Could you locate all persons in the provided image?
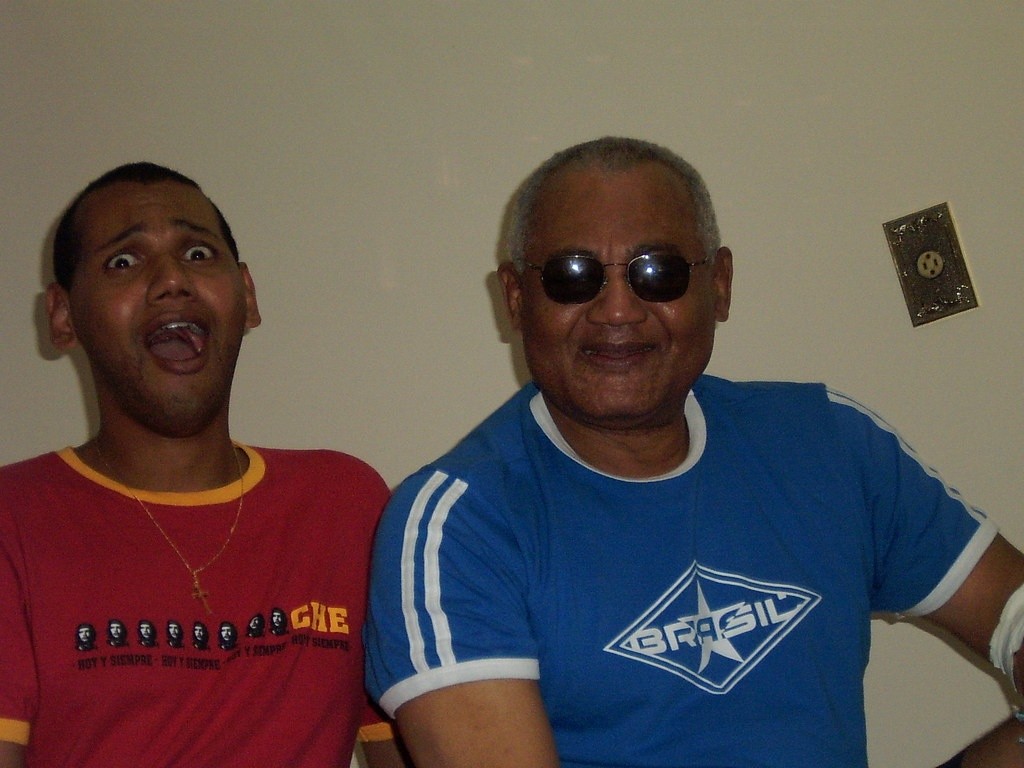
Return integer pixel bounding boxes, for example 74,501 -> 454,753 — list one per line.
1,159 -> 416,767
370,136 -> 1024,768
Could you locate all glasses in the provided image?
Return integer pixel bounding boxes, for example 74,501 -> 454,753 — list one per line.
522,249 -> 712,303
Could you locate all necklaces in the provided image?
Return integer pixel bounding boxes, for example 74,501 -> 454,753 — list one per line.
93,431 -> 247,614
